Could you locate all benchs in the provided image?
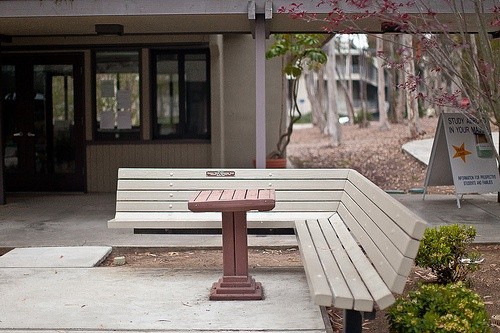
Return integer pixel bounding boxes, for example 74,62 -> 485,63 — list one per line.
108,165 -> 427,333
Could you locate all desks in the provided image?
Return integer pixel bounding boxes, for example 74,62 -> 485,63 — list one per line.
187,184 -> 276,301
